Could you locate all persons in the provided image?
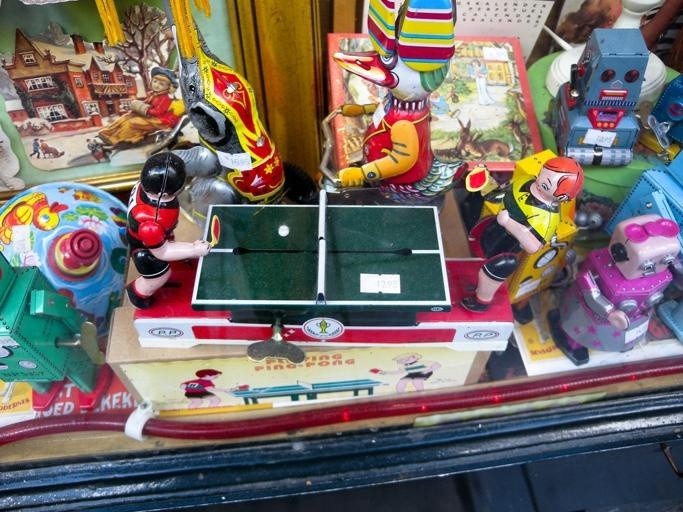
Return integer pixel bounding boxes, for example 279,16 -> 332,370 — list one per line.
459,156 -> 584,313
126,148 -> 210,309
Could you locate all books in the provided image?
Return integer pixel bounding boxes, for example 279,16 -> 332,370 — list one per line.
327,32 -> 543,189
